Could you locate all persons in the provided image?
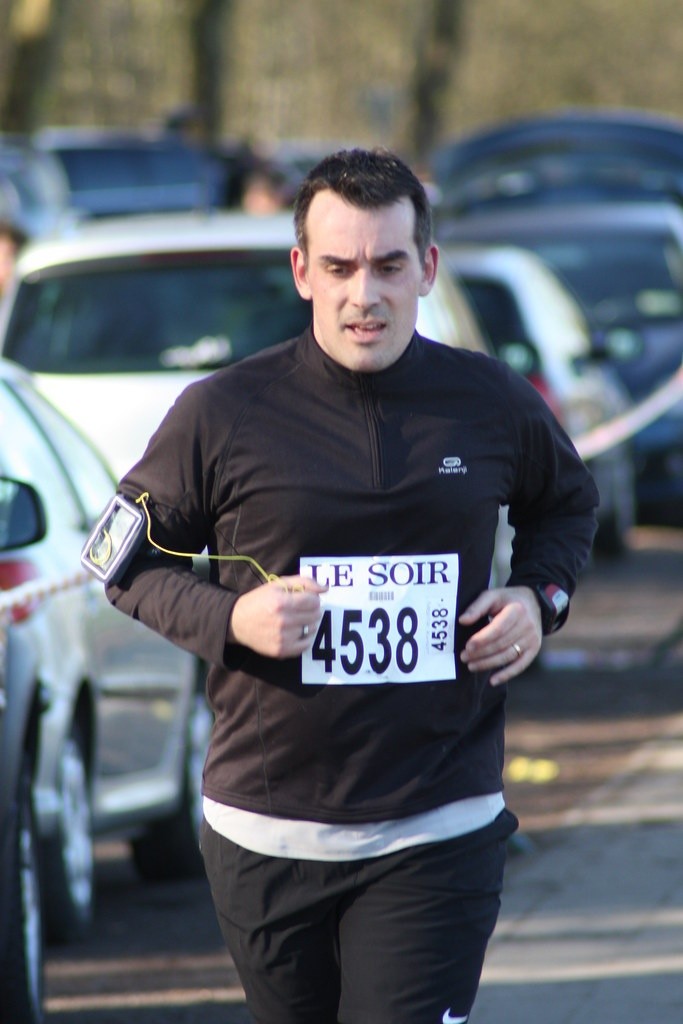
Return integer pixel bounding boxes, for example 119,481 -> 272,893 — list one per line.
79,148 -> 603,1024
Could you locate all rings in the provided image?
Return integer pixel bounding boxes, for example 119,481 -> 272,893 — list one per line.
513,644 -> 521,656
301,626 -> 310,639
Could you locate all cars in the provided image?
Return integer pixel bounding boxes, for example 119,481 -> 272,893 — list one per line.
1,475 -> 60,1024
0,105 -> 683,600
1,352 -> 216,948
